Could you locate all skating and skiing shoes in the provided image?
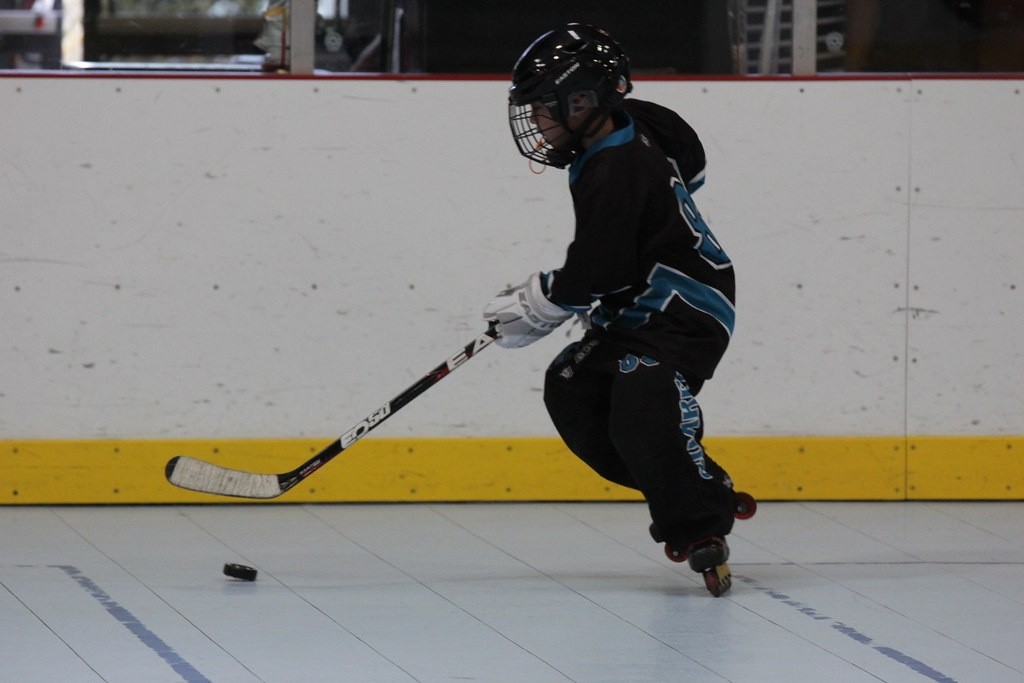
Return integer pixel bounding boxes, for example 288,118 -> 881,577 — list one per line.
686,536 -> 727,597
650,468 -> 756,562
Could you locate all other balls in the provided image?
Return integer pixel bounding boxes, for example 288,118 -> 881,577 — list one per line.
223,562 -> 258,582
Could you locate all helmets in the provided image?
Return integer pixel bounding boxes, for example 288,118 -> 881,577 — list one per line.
508,23 -> 633,169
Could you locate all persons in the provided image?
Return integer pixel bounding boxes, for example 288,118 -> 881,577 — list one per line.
481,22 -> 757,598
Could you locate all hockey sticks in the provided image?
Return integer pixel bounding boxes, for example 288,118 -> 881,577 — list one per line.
165,320 -> 498,499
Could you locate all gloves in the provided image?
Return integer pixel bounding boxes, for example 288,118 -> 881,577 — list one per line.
483,272 -> 574,348
